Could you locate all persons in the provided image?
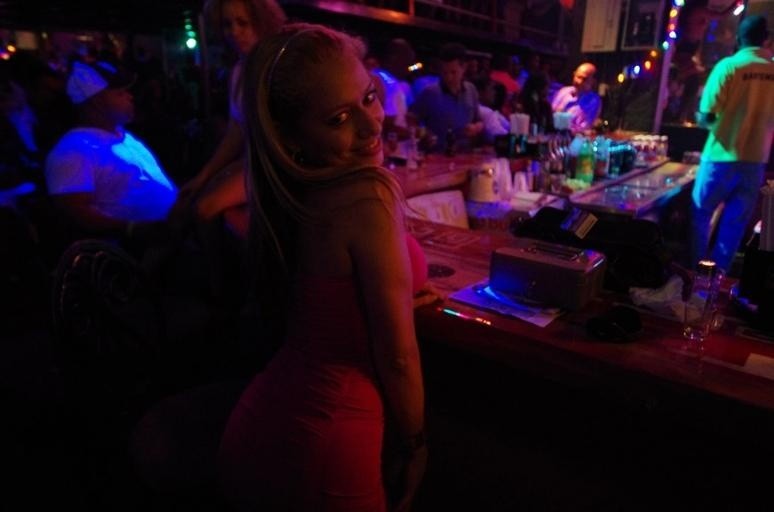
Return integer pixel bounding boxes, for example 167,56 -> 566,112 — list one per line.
217,20 -> 427,510
691,13 -> 772,272
43,58 -> 183,455
1,1 -> 704,350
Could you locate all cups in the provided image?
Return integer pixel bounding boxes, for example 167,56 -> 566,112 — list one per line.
684,302 -> 717,341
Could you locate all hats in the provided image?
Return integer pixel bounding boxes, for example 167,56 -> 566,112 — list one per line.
65,58 -> 137,105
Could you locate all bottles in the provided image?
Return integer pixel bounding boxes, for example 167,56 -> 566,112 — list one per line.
688,252 -> 743,334
522,133 -> 609,190
